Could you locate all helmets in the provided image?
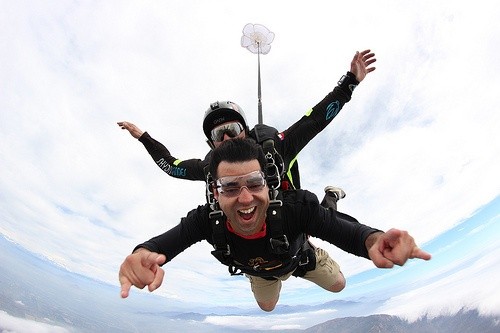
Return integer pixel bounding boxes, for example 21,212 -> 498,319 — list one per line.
204,99 -> 250,147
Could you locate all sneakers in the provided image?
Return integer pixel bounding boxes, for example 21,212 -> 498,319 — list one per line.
324,186 -> 346,199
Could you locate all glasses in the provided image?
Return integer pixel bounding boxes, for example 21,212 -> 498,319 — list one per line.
210,169 -> 269,198
209,123 -> 247,144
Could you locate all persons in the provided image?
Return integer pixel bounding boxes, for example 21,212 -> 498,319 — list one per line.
116,47 -> 376,210
119,138 -> 431,311
